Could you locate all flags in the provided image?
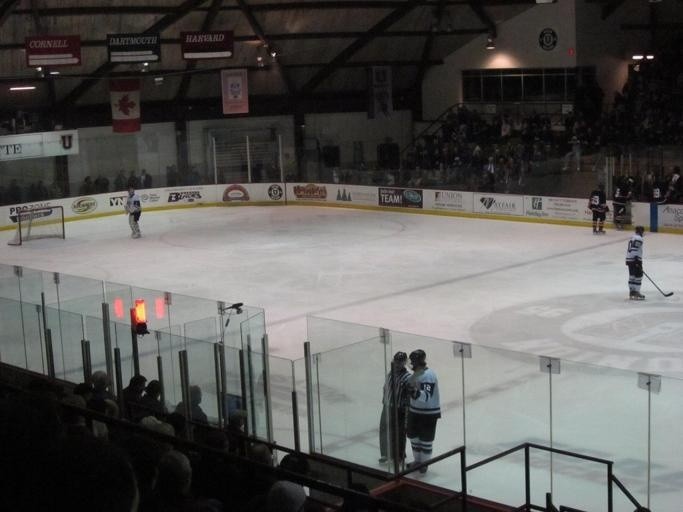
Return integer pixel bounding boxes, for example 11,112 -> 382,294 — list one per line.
220,69 -> 249,115
107,77 -> 140,135
179,29 -> 235,61
106,31 -> 163,66
23,35 -> 83,73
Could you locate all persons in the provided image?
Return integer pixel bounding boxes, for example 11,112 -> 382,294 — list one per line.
0,357 -> 315,512
123,187 -> 141,239
588,181 -> 610,237
0,154 -> 297,207
405,349 -> 441,474
625,225 -> 647,299
377,351 -> 411,464
613,174 -> 635,229
331,46 -> 683,205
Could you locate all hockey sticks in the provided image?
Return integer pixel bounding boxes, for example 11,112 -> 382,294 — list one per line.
642,269 -> 673,297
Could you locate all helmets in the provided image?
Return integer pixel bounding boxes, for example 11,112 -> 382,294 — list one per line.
395,351 -> 407,362
636,225 -> 644,234
597,182 -> 605,187
410,349 -> 426,364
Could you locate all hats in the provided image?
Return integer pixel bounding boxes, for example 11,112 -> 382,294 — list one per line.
229,410 -> 248,420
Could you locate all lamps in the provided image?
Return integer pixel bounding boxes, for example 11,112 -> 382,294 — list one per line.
483,35 -> 497,50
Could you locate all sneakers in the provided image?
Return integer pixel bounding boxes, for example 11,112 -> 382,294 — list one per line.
407,461 -> 427,474
378,456 -> 393,463
630,291 -> 645,298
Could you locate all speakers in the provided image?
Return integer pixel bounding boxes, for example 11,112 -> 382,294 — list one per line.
324,146 -> 340,166
377,143 -> 400,169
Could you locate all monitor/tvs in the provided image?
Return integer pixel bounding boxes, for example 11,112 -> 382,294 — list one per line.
221,392 -> 244,424
